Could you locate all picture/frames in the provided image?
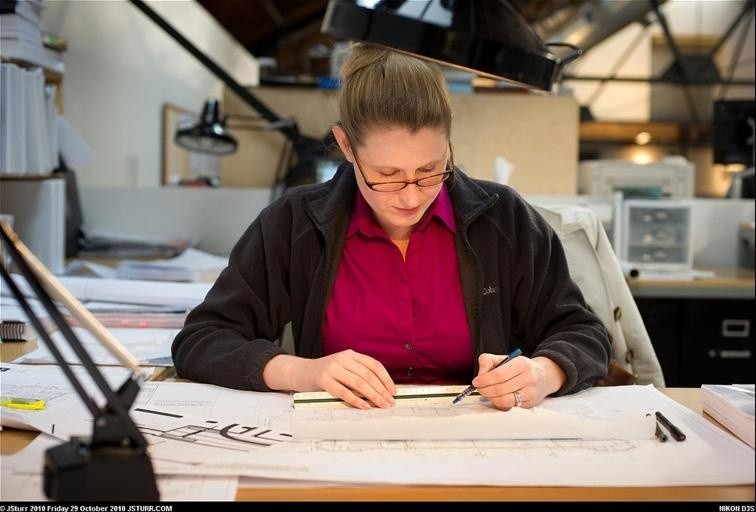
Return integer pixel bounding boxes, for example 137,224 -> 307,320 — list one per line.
621,199 -> 697,273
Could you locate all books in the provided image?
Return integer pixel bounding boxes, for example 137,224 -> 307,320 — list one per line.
59,301 -> 194,330
0,295 -> 62,343
1,0 -> 77,279
79,222 -> 203,262
109,259 -> 207,284
698,381 -> 756,450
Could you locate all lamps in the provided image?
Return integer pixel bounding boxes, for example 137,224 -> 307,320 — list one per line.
173,98 -> 301,203
318,0 -> 587,99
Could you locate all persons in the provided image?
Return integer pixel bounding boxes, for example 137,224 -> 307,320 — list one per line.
169,37 -> 615,413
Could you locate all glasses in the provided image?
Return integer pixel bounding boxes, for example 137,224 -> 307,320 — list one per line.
336,120 -> 454,193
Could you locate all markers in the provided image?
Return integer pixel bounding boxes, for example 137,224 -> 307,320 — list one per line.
0,396 -> 46,410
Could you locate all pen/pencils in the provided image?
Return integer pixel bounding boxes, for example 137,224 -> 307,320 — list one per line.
449,348 -> 522,407
646,412 -> 685,442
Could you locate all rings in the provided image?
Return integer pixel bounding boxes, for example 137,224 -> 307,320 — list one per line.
511,391 -> 522,408
